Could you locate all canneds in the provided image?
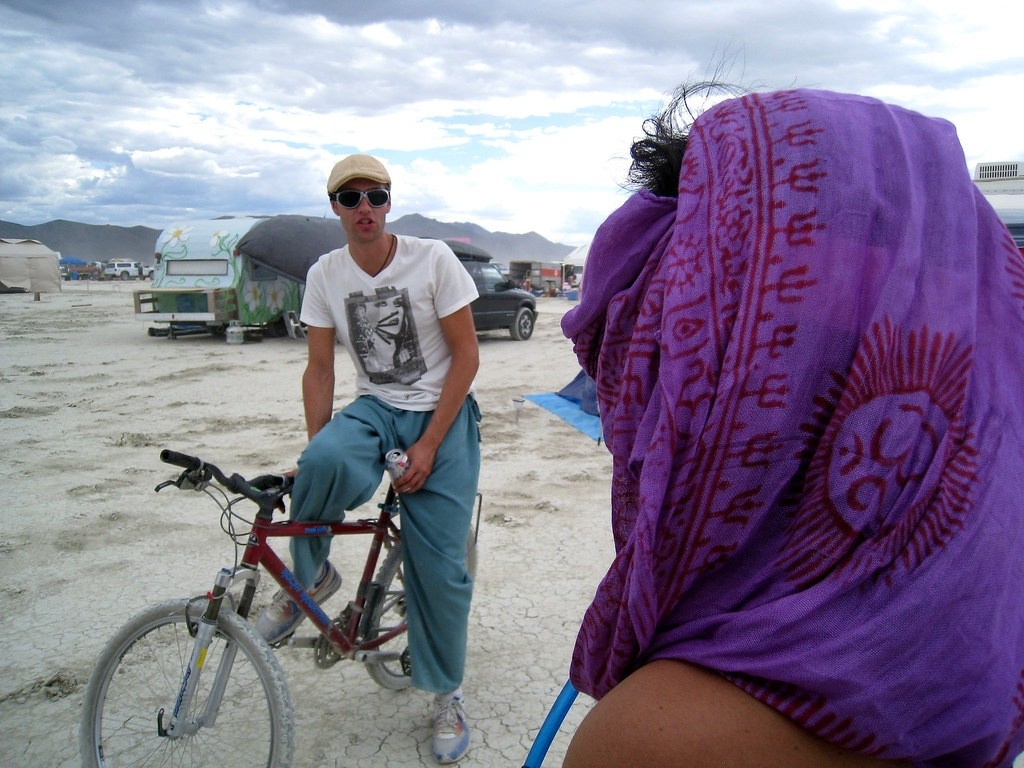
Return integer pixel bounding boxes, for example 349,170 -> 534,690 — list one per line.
384,449 -> 410,490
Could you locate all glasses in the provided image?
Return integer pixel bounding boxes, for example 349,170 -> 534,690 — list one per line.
329,189 -> 390,208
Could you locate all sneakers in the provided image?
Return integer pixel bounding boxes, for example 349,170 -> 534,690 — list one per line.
430,692 -> 470,763
252,558 -> 342,644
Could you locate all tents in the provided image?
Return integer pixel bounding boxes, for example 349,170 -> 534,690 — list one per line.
0,238 -> 63,294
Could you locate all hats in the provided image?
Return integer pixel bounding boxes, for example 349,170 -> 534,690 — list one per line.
327,154 -> 391,193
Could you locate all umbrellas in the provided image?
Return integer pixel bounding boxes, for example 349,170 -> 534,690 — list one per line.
563,239 -> 593,266
59,255 -> 87,265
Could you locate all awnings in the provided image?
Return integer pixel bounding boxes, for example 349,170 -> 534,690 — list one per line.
442,240 -> 494,263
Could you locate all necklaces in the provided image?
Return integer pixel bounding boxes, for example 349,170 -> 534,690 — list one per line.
372,234 -> 394,277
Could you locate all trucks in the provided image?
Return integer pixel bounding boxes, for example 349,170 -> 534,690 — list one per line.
510,260 -> 575,292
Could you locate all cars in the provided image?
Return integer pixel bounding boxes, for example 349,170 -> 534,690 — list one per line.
572,265 -> 583,286
104,262 -> 154,281
420,236 -> 538,340
482,262 -> 509,285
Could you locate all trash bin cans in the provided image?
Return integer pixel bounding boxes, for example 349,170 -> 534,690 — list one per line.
545,280 -> 558,297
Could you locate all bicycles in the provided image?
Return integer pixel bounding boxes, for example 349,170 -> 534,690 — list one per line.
78,450 -> 482,768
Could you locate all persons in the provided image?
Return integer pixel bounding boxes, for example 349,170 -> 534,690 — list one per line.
90,269 -> 98,281
564,87 -> 1023,767
64,261 -> 71,280
561,267 -> 574,282
138,263 -> 143,281
110,269 -> 117,280
252,153 -> 482,764
